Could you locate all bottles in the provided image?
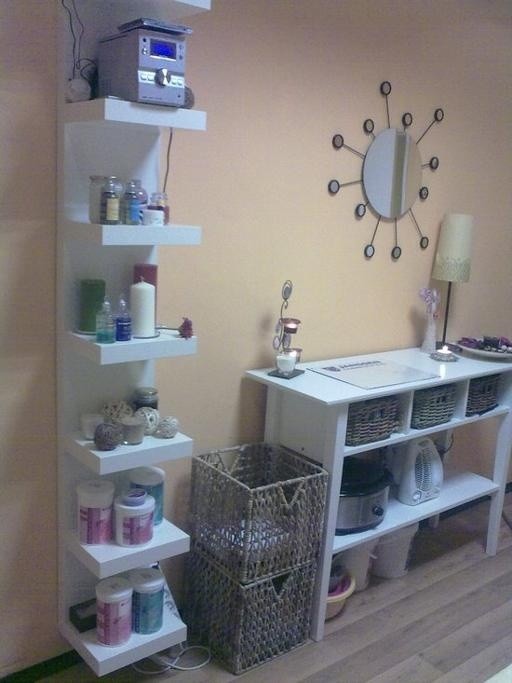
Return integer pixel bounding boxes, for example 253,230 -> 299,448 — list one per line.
114,299 -> 131,341
75,465 -> 166,549
128,568 -> 165,635
95,578 -> 133,647
94,295 -> 117,344
132,387 -> 159,412
99,175 -> 171,227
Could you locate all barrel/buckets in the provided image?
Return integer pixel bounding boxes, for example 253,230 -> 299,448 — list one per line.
333,538 -> 381,594
371,522 -> 422,580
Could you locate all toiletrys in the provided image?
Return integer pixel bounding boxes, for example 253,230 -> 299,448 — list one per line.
112,297 -> 132,342
95,301 -> 117,343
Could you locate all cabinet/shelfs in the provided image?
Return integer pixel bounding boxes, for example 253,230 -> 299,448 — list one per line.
54,0 -> 211,675
263,349 -> 510,644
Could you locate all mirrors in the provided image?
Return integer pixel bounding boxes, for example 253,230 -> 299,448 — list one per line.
361,127 -> 423,221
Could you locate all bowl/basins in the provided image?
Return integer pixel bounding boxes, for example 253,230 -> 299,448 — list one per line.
325,563 -> 356,623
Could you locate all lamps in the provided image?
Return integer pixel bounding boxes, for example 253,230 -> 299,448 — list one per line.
426,212 -> 479,362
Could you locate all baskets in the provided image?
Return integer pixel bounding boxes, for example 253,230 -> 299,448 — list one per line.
411,384 -> 458,430
466,374 -> 502,417
184,548 -> 318,676
344,395 -> 400,447
186,440 -> 329,585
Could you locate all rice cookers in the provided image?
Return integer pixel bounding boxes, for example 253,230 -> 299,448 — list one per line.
336,456 -> 395,536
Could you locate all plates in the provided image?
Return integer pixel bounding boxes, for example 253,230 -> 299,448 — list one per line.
267,369 -> 306,380
452,338 -> 512,359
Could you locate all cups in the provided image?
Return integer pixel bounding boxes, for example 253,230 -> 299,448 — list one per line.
134,263 -> 158,302
79,414 -> 104,439
78,278 -> 106,333
119,415 -> 145,445
276,354 -> 296,374
